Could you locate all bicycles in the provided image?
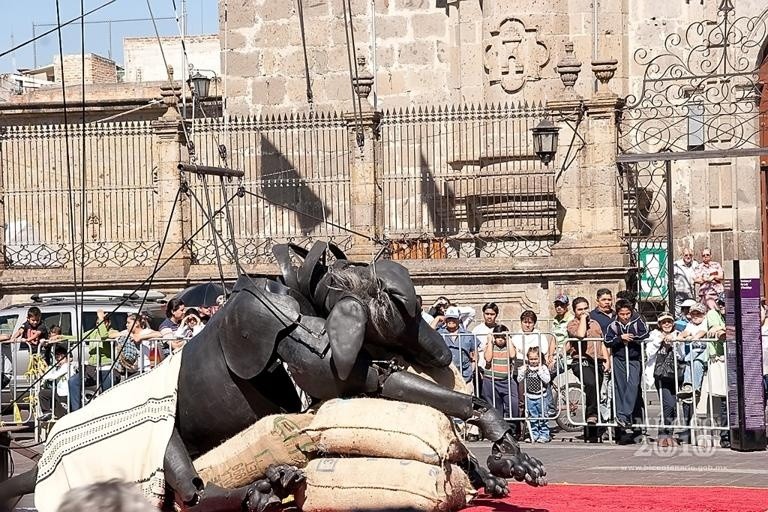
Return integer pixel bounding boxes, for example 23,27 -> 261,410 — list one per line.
549,358 -> 588,433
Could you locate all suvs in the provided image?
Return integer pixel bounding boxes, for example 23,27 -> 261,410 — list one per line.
0,288 -> 170,411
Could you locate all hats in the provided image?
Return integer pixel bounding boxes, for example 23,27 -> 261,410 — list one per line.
553,295 -> 568,303
445,306 -> 460,319
657,299 -> 706,322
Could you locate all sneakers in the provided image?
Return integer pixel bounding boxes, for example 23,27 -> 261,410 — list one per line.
524,437 -> 547,443
677,385 -> 693,399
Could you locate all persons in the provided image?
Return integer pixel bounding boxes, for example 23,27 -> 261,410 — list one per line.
416,248 -> 768,445
1,294 -> 228,422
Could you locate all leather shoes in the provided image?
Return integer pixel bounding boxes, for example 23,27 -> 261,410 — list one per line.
587,417 -> 596,424
37,414 -> 51,421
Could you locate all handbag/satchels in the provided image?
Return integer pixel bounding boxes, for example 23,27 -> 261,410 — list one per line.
654,340 -> 685,382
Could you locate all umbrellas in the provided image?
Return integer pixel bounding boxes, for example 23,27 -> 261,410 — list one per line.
173,277 -> 231,306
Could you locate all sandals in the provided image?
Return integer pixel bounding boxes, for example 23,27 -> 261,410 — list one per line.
615,416 -> 627,428
